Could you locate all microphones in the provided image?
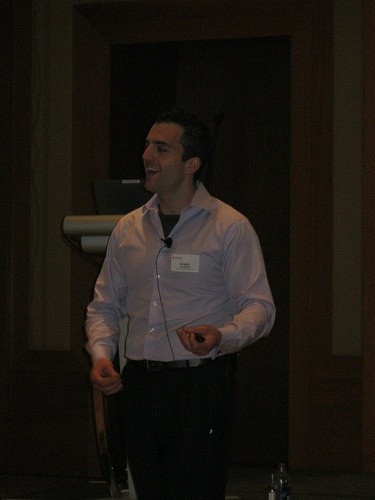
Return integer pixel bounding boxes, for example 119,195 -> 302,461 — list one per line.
161,238 -> 173,248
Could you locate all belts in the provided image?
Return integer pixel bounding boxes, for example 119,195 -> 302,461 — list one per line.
132,360 -> 187,369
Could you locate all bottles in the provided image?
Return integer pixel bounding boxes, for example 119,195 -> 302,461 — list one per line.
271,463 -> 288,500
268,489 -> 276,500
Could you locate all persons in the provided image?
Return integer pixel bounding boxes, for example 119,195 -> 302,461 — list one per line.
84,112 -> 277,500
